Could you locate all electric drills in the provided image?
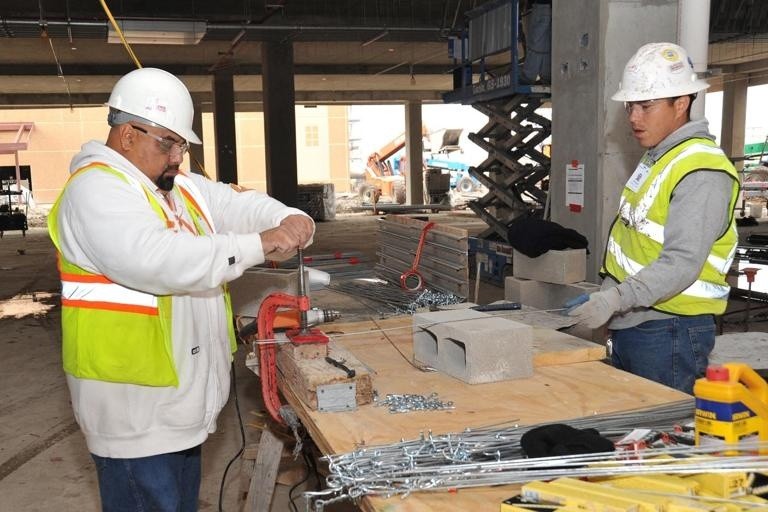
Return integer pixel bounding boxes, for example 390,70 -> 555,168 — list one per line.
240,310 -> 357,338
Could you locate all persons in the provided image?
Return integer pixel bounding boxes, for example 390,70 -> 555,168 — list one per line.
45,67 -> 317,512
565,40 -> 741,399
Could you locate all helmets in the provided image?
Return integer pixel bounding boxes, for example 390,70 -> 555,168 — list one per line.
610,42 -> 711,102
104,68 -> 202,146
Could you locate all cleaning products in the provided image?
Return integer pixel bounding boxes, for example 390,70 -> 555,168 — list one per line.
693,362 -> 768,457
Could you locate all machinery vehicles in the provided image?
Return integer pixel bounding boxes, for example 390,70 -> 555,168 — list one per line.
392,151 -> 483,193
360,120 -> 466,215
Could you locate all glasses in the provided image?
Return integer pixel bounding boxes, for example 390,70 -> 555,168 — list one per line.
624,99 -> 673,114
132,125 -> 190,155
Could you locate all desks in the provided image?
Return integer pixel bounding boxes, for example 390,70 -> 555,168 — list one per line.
223,277 -> 700,512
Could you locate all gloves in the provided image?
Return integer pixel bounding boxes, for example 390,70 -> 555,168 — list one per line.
567,286 -> 621,329
